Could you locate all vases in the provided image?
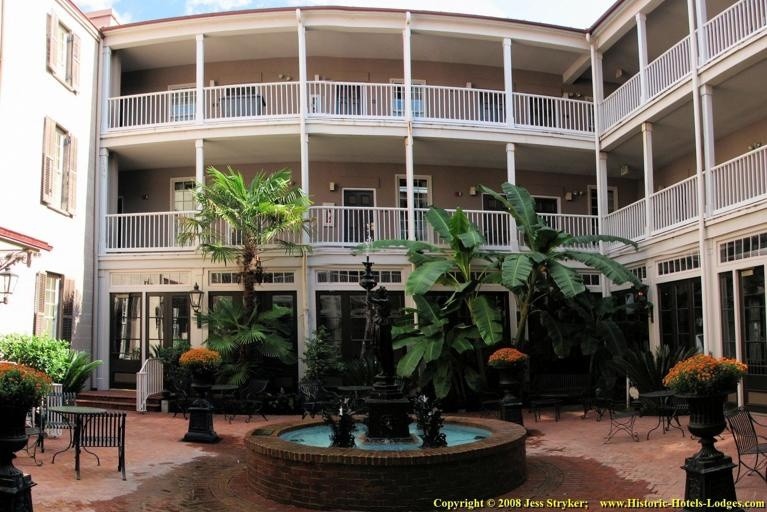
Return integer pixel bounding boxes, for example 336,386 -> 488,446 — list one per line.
183,368 -> 223,444
0,414 -> 33,511
496,361 -> 528,434
674,393 -> 745,512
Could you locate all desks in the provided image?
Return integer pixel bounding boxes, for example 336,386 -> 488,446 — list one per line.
338,385 -> 374,408
639,389 -> 685,440
209,384 -> 239,419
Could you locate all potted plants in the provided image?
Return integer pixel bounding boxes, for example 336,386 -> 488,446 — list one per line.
0,362 -> 50,414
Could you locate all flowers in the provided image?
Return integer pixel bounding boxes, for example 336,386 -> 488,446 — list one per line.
663,352 -> 748,396
178,348 -> 222,374
488,345 -> 529,366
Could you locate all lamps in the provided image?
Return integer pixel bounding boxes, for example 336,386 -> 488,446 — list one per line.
0,247 -> 41,304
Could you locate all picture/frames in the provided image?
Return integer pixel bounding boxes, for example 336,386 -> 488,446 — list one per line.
321,202 -> 336,228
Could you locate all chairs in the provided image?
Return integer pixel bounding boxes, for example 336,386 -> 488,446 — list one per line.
171,376 -> 194,420
298,382 -> 344,420
725,405 -> 767,485
26,390 -> 127,481
227,379 -> 270,423
531,367 -> 640,442
